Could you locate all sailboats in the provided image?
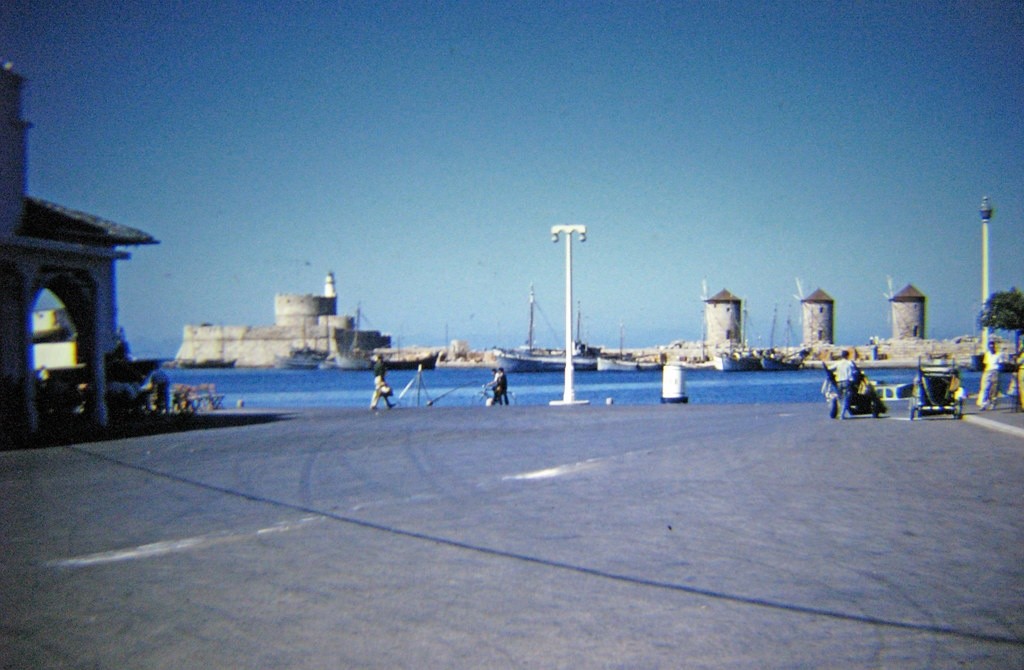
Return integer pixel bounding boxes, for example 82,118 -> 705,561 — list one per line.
713,295 -> 737,371
735,299 -> 762,370
334,303 -> 372,371
760,302 -> 811,370
493,284 -> 596,371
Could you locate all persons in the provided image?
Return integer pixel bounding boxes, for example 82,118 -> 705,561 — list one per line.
1013,334 -> 1024,412
150,366 -> 171,414
821,350 -> 858,421
486,366 -> 511,406
976,342 -> 1003,412
371,354 -> 397,409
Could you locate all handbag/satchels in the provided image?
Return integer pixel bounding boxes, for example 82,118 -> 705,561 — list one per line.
379,386 -> 393,397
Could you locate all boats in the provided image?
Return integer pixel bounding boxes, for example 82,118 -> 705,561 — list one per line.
680,361 -> 714,368
871,383 -> 914,401
596,358 -> 636,372
638,361 -> 660,370
179,357 -> 236,368
373,351 -> 437,369
276,344 -> 330,369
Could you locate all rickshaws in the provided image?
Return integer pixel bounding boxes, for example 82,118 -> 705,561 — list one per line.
908,355 -> 964,421
822,358 -> 884,418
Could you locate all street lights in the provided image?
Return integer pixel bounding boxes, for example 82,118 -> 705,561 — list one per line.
979,195 -> 991,352
550,223 -> 587,403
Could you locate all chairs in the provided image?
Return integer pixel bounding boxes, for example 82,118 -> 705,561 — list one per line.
173,384 -> 225,410
